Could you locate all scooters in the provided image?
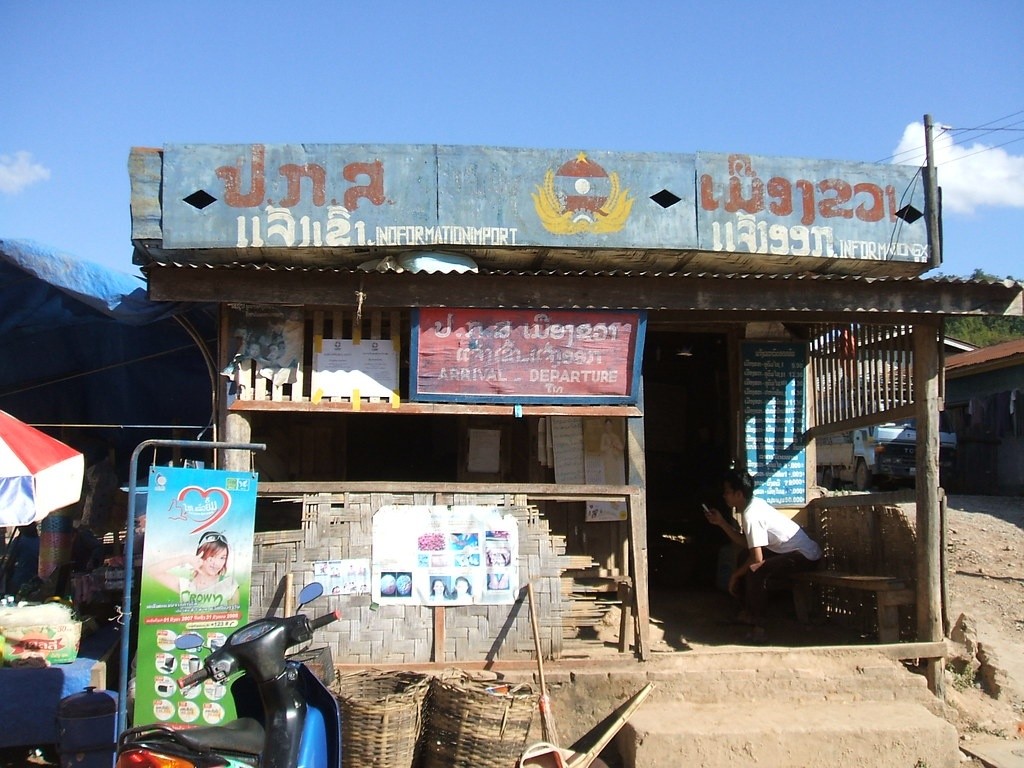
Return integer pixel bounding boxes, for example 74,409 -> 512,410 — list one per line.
114,580 -> 341,768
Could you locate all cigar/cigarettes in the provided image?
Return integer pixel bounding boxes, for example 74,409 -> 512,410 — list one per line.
702,503 -> 712,514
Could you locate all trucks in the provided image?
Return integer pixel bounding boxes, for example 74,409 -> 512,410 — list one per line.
816,398 -> 958,491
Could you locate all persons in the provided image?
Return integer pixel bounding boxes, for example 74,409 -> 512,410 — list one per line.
428,576 -> 475,600
147,531 -> 242,620
701,470 -> 826,644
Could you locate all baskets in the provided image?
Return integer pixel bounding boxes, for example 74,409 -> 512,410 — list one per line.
285,646 -> 335,687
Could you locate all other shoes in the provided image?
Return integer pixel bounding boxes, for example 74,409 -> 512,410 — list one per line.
730,613 -> 769,644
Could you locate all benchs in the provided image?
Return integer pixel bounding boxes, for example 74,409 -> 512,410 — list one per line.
775,570 -> 916,645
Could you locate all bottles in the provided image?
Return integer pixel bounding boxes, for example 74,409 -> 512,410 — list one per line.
64,595 -> 81,622
0,593 -> 17,607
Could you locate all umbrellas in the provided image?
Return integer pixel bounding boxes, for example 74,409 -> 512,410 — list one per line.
0,406 -> 85,531
